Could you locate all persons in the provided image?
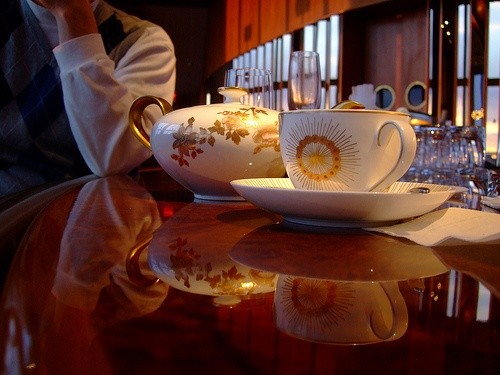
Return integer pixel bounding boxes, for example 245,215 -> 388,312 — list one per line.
0,1 -> 177,189
4,177 -> 170,375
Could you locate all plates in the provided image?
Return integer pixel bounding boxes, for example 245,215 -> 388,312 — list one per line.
230,221 -> 454,283
230,177 -> 469,229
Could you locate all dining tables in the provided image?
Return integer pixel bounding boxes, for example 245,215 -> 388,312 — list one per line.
0,167 -> 500,375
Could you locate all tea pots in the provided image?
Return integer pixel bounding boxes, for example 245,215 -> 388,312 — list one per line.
129,86 -> 287,206
127,200 -> 284,308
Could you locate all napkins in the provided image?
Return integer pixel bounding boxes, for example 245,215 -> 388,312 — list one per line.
361,208 -> 500,247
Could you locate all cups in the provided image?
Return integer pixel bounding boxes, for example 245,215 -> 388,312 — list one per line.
289,50 -> 322,110
274,275 -> 412,345
224,68 -> 274,111
277,109 -> 418,192
414,125 -> 484,210
